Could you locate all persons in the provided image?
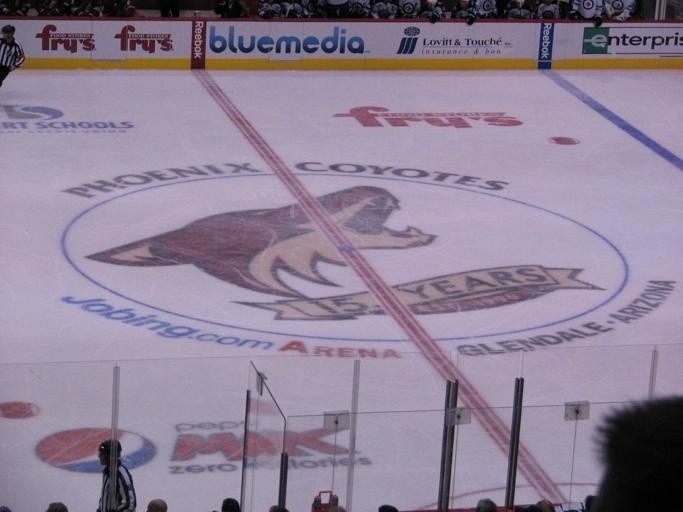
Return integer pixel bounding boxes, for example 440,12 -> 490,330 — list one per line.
145,498 -> 167,511
220,496 -> 239,510
0,25 -> 25,88
0,0 -> 683,26
96,439 -> 137,512
585,391 -> 682,510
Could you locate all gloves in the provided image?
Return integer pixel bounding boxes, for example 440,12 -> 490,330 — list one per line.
430,13 -> 440,24
592,16 -> 603,28
466,13 -> 477,26
605,4 -> 615,19
259,9 -> 278,20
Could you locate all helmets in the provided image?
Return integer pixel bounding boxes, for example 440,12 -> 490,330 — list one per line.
2,25 -> 16,34
100,438 -> 122,458
312,490 -> 338,510
